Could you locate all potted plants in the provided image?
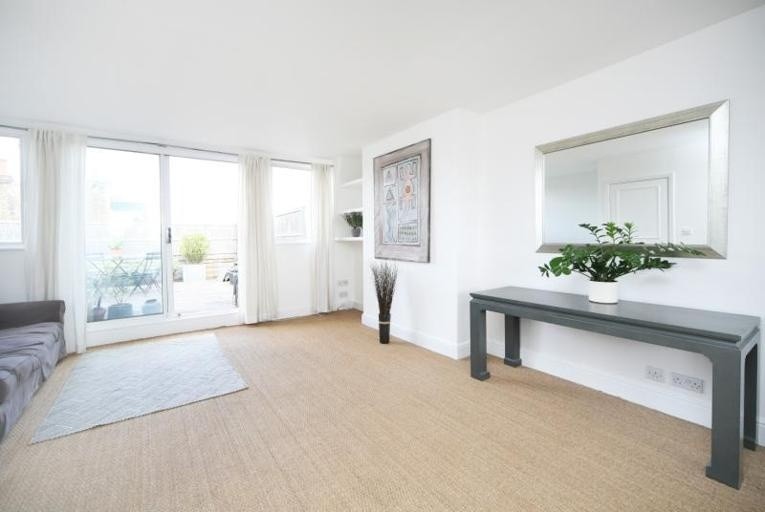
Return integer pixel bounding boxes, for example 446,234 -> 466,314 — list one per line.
89,278 -> 106,321
340,211 -> 363,237
105,273 -> 133,319
538,219 -> 708,305
178,233 -> 210,290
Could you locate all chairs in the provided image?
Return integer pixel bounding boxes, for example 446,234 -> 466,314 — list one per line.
129,251 -> 161,297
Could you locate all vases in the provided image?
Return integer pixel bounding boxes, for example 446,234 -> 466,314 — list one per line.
378,313 -> 390,345
141,299 -> 161,315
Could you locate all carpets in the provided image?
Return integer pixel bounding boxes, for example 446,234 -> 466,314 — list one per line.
26,330 -> 250,445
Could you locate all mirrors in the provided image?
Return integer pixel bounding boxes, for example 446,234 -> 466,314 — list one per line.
533,99 -> 728,260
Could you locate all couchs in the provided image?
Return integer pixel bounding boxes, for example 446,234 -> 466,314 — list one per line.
0,301 -> 67,442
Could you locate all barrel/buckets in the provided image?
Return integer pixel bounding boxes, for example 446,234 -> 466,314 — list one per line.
182,264 -> 207,289
87,308 -> 106,321
107,302 -> 133,320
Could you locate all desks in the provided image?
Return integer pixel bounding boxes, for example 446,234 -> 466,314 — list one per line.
467,285 -> 761,490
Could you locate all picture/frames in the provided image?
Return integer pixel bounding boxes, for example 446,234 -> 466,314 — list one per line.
372,138 -> 431,265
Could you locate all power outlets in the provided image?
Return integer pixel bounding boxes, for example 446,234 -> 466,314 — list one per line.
670,371 -> 704,394
643,365 -> 667,383
